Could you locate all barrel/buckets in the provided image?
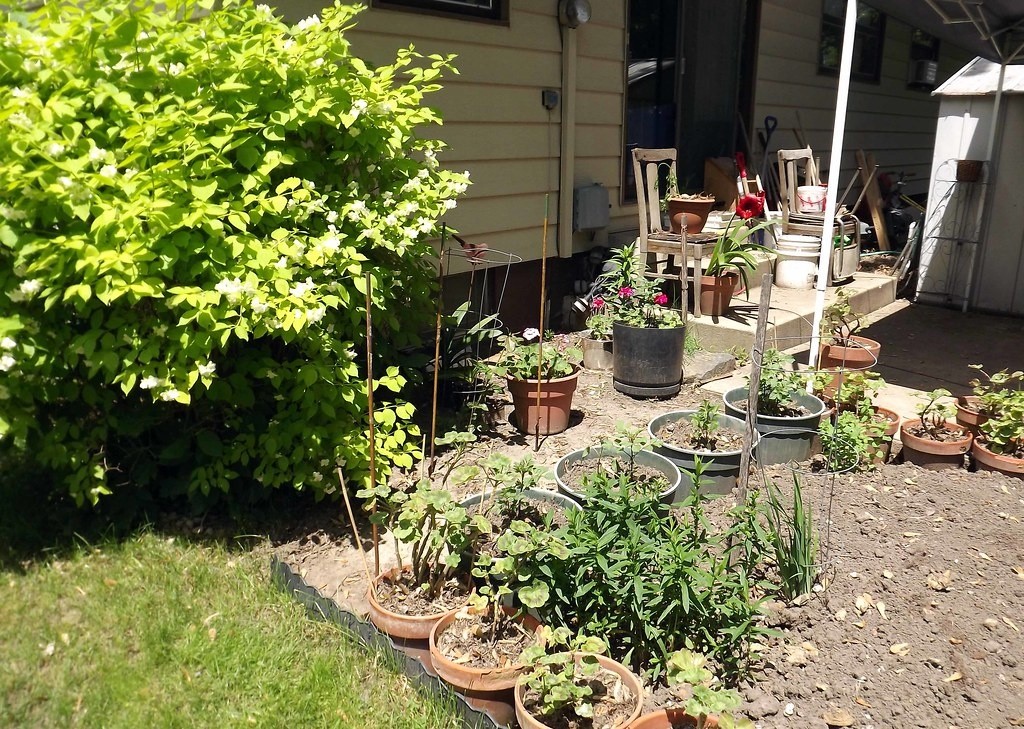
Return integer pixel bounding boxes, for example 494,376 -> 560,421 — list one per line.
798,185 -> 827,212
774,233 -> 822,288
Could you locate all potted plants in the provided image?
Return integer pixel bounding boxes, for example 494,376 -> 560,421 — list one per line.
651,163 -> 714,233
368,290 -> 1024,729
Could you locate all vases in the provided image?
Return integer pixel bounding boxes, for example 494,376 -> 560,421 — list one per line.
612,319 -> 687,395
578,330 -> 614,375
699,271 -> 738,317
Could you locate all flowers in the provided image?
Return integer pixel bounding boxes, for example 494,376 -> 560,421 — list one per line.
704,193 -> 781,300
583,300 -> 614,340
620,289 -> 682,327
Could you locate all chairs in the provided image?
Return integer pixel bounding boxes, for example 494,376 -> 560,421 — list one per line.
633,148 -> 728,318
778,148 -> 860,286
